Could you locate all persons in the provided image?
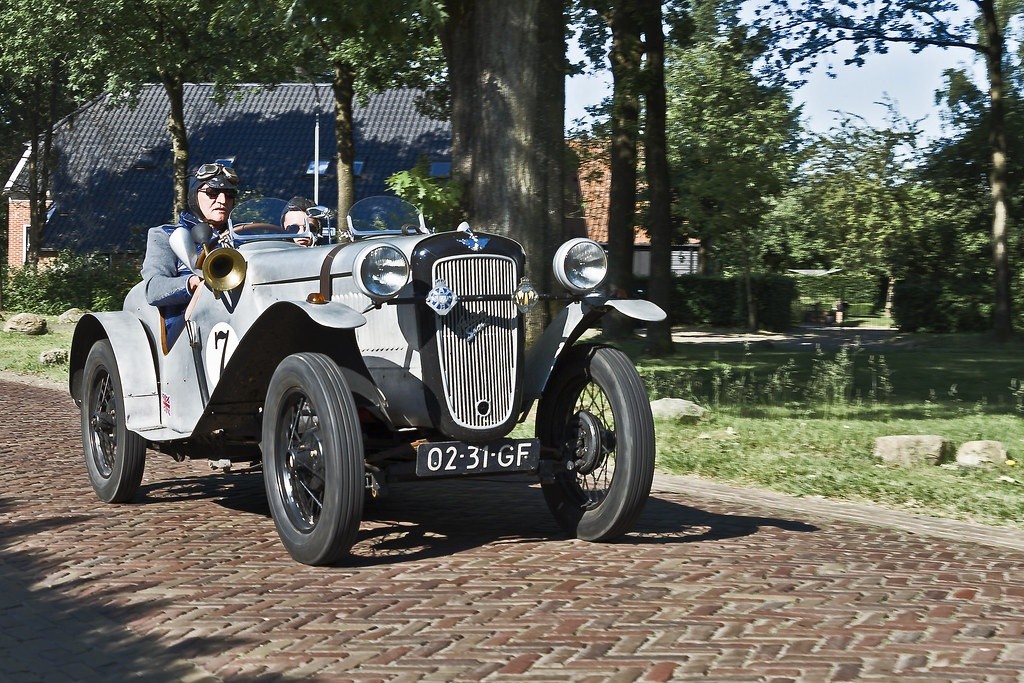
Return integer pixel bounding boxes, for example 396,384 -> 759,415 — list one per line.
140,162 -> 239,352
280,195 -> 325,247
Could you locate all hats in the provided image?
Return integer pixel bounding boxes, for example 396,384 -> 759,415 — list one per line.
188,173 -> 237,219
281,198 -> 322,240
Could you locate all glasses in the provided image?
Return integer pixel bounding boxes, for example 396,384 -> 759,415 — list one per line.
195,164 -> 240,184
197,188 -> 238,199
286,223 -> 317,234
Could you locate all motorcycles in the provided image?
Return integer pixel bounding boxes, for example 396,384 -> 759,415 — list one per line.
67,196 -> 668,566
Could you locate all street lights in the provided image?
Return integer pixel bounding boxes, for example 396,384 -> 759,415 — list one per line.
293,66 -> 322,207
